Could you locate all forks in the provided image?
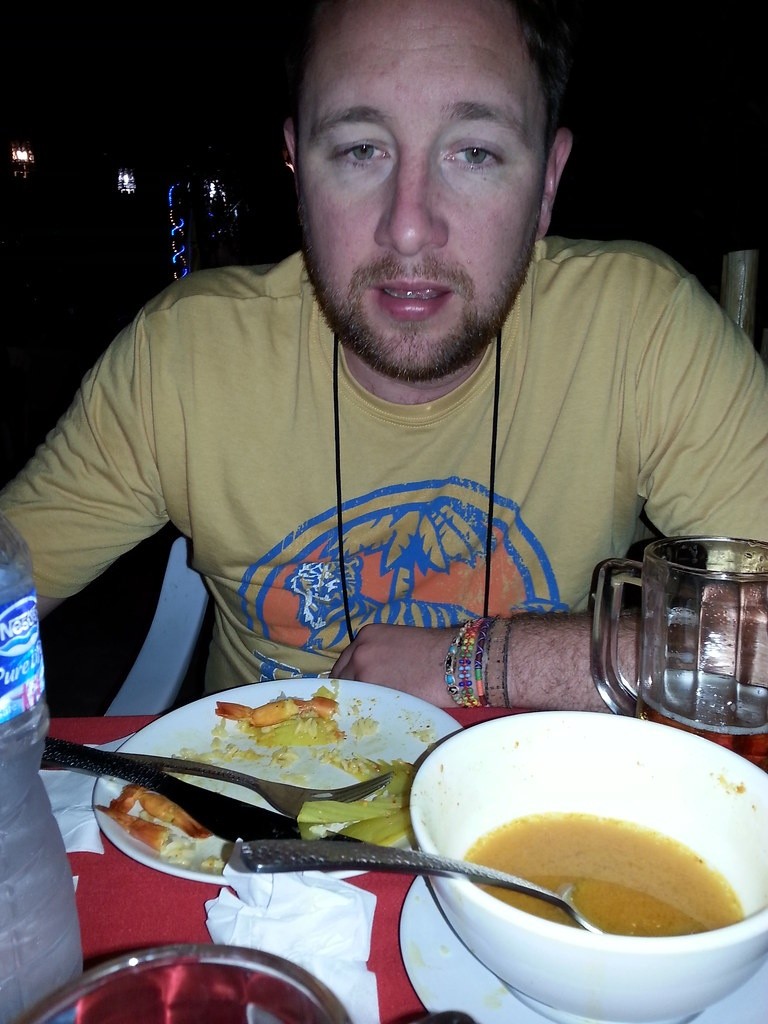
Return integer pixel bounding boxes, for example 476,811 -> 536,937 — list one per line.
40,751 -> 394,818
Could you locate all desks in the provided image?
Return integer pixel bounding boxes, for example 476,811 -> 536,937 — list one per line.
39,706 -> 768,1024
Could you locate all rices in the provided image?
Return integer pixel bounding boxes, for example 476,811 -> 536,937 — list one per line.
139,680 -> 438,869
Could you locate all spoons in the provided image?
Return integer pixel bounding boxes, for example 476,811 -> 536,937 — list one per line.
222,837 -> 607,935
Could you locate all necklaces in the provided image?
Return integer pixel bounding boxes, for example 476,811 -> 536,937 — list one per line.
332,333 -> 501,644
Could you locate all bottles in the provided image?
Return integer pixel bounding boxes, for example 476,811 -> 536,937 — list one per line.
0,508 -> 83,1024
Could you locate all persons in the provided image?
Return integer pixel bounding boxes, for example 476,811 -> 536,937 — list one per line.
0,1 -> 768,712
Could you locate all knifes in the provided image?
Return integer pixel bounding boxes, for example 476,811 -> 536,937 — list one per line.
42,737 -> 364,843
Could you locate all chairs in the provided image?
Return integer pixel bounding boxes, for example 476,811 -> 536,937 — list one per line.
104,536 -> 210,717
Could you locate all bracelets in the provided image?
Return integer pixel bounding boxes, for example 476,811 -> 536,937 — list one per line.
442,616 -> 511,708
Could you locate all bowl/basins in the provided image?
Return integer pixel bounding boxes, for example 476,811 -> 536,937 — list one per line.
410,712 -> 768,1024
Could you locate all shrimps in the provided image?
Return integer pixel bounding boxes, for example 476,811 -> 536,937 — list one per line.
94,784 -> 212,852
215,696 -> 340,727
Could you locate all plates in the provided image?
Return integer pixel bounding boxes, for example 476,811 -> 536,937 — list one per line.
399,872 -> 768,1024
92,678 -> 466,887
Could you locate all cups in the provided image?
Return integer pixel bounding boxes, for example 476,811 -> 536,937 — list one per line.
30,943 -> 481,1024
588,534 -> 768,771
